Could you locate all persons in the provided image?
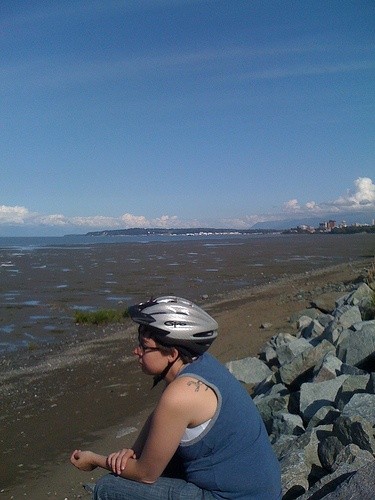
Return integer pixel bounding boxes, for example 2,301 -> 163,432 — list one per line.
69,296 -> 284,500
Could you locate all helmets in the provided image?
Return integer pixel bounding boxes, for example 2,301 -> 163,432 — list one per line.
128,295 -> 219,354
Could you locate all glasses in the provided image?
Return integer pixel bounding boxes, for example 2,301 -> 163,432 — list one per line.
138,339 -> 172,356
